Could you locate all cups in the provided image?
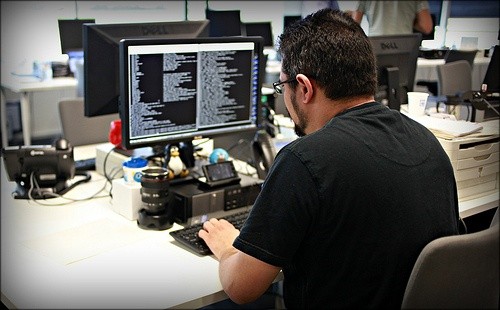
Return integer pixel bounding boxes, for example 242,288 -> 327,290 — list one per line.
407,92 -> 430,118
121,158 -> 171,214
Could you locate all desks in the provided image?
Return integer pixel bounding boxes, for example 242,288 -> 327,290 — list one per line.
1,128 -> 499,309
413,53 -> 490,123
0,65 -> 78,146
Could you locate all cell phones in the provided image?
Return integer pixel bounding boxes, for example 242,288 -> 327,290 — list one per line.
202,161 -> 238,183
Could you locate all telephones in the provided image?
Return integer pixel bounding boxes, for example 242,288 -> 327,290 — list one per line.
2,137 -> 76,199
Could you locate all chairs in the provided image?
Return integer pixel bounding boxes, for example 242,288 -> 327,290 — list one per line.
401,208 -> 499,310
58,99 -> 119,146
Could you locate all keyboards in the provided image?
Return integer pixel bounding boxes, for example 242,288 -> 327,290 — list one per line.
76,158 -> 96,171
169,211 -> 250,256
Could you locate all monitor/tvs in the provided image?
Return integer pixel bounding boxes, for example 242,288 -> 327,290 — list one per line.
367,32 -> 422,111
59,19 -> 274,183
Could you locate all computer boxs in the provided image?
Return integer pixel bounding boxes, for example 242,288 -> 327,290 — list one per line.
162,158 -> 265,227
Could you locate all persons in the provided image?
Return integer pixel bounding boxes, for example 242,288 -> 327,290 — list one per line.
199,7 -> 459,310
344,0 -> 433,35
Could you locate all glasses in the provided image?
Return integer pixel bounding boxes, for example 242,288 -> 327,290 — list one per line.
273,77 -> 296,94
